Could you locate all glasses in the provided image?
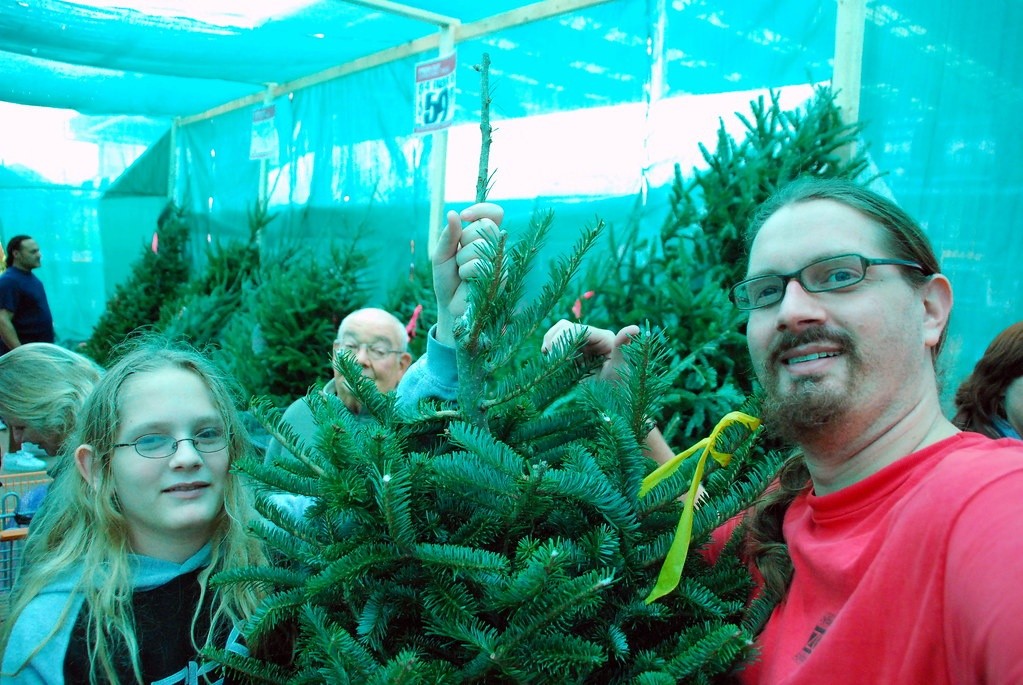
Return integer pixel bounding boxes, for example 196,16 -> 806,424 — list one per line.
338,341 -> 404,359
113,427 -> 235,459
727,253 -> 929,310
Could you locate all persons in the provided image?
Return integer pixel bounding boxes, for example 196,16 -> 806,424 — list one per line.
264,307 -> 411,492
951,321 -> 1023,441
1,203 -> 505,684
0,342 -> 110,460
0,235 -> 57,351
539,173 -> 1023,683
